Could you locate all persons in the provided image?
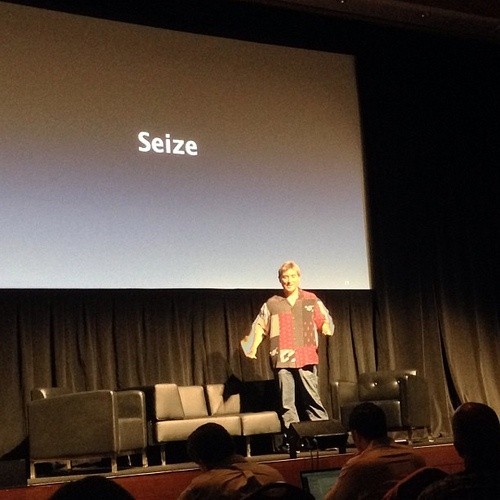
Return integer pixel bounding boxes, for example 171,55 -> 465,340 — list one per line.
326,402 -> 425,500
418,402 -> 500,500
48,477 -> 136,500
240,262 -> 334,450
178,423 -> 285,500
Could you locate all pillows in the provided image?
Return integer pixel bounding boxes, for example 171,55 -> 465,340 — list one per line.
152,384 -> 186,421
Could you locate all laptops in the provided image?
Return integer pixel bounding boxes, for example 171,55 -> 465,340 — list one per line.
300,467 -> 341,500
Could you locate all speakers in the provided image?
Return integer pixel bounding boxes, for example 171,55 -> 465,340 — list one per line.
281,419 -> 349,459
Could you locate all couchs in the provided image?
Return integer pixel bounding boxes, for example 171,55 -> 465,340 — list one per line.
147,380 -> 283,467
335,366 -> 433,448
24,385 -> 149,479
